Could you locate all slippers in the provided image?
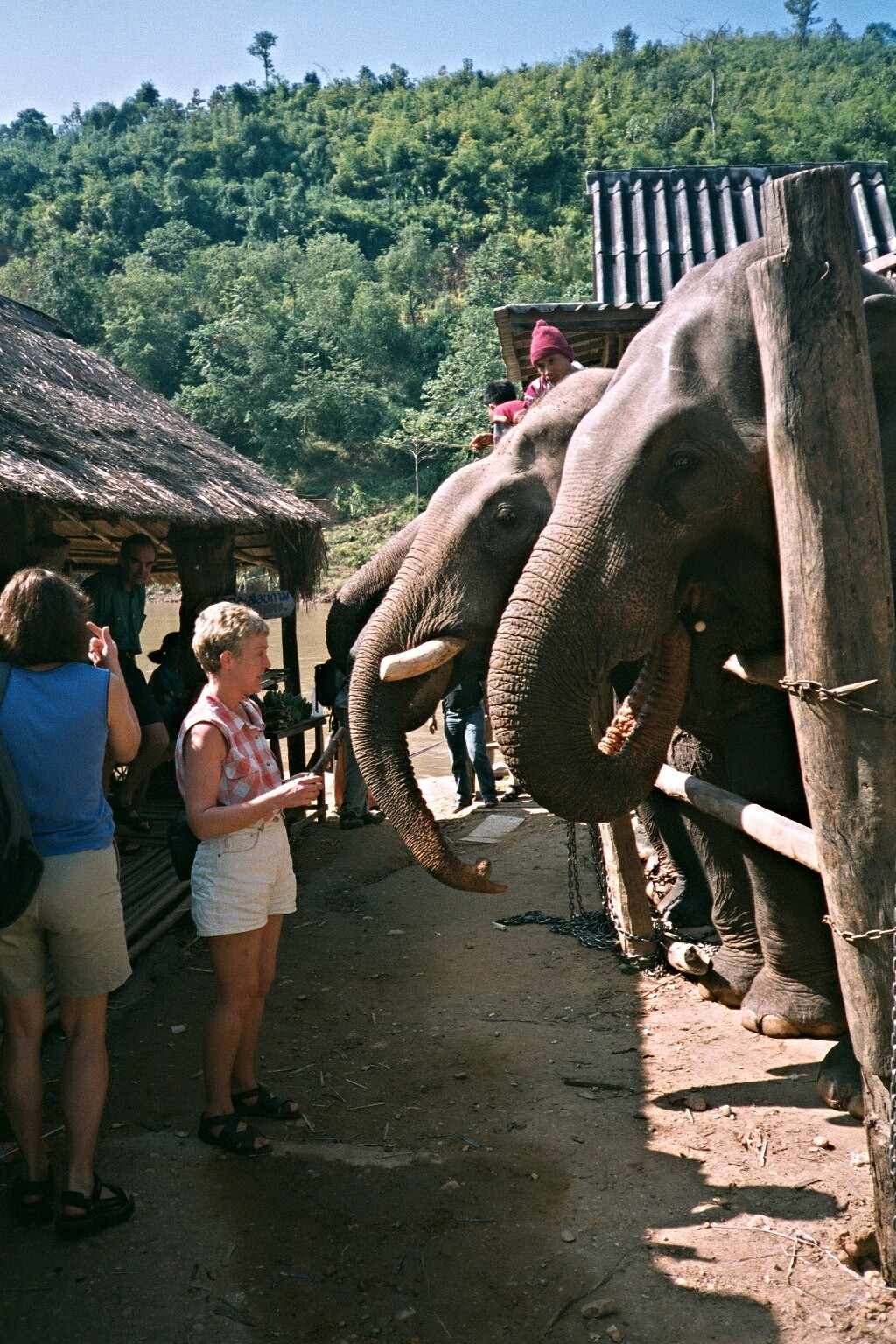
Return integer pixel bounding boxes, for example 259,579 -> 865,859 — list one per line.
12,1161 -> 58,1225
197,1110 -> 273,1157
60,1171 -> 136,1238
231,1082 -> 303,1119
451,801 -> 472,813
117,803 -> 150,838
483,791 -> 498,808
117,838 -> 143,854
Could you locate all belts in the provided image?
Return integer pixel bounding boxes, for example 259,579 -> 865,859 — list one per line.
447,701 -> 480,714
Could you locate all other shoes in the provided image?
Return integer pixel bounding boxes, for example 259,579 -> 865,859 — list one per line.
339,812 -> 363,829
360,811 -> 383,825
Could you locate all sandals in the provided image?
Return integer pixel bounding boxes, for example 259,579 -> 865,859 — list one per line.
501,784 -> 524,802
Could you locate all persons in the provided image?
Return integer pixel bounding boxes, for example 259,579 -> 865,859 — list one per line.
0,569 -> 142,1241
332,684 -> 385,829
79,534 -> 169,857
147,632 -> 179,709
176,602 -> 324,1155
427,679 -> 499,814
469,379 -> 517,450
493,319 -> 582,446
500,771 -> 524,802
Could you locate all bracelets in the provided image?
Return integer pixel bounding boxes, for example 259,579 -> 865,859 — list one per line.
431,715 -> 435,718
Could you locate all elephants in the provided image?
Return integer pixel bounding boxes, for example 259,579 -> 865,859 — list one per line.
484,241 -> 896,1122
336,360 -> 774,899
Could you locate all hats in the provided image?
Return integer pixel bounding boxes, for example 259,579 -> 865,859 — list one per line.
147,632 -> 194,665
530,319 -> 575,368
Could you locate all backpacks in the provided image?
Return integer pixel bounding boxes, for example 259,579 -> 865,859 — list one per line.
312,659 -> 350,713
0,660 -> 45,931
